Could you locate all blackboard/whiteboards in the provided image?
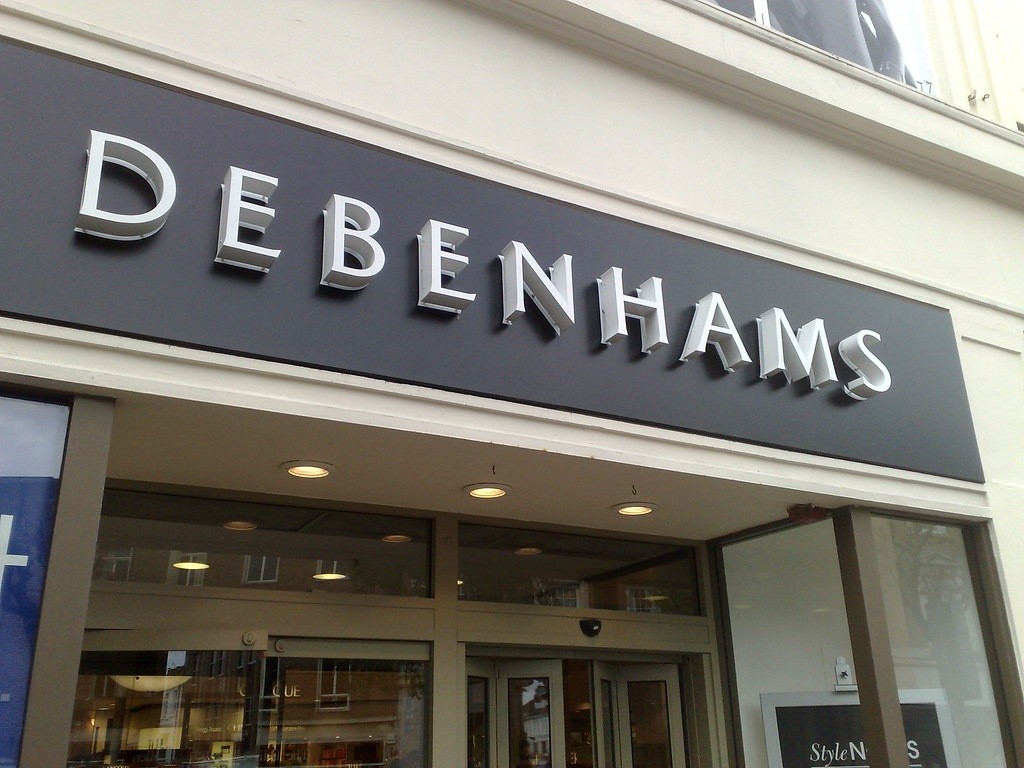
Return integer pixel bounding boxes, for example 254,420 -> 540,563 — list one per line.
758,688 -> 962,768
